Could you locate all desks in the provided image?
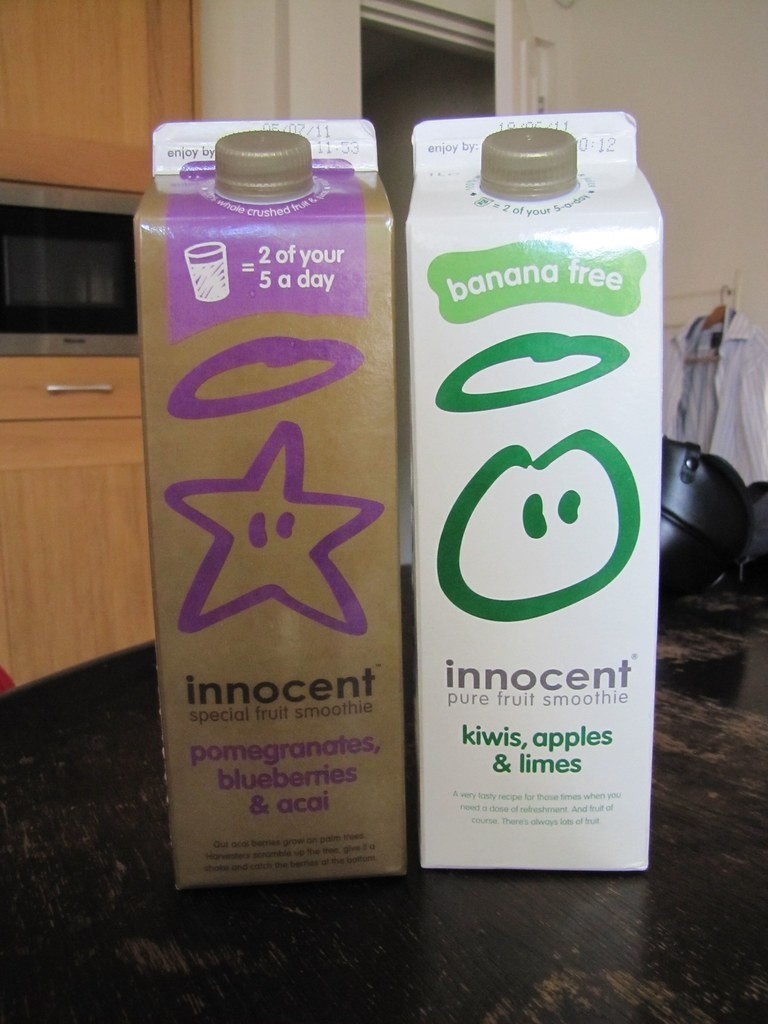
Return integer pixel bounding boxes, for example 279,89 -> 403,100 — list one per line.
0,590 -> 768,1024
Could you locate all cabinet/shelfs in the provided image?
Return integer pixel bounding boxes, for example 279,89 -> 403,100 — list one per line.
0,0 -> 206,694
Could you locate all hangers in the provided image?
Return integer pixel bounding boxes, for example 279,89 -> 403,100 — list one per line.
686,285 -> 732,361
